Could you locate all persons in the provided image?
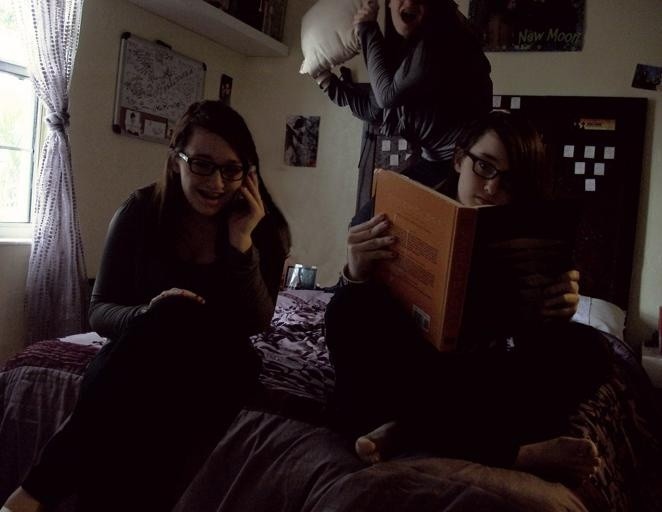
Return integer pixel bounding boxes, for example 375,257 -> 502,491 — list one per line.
326,110 -> 601,484
0,101 -> 292,512
312,0 -> 493,226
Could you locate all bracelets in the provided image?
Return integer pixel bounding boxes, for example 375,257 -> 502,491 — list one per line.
316,71 -> 332,89
342,263 -> 372,284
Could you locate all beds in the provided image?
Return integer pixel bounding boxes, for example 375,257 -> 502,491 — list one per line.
1,285 -> 628,510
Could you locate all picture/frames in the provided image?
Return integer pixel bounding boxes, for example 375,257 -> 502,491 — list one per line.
284,266 -> 294,287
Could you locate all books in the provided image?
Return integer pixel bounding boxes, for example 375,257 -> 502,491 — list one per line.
372,167 -> 579,353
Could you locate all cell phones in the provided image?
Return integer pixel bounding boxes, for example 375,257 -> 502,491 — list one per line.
235,165 -> 256,213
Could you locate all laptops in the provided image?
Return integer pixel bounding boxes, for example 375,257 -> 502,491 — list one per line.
283,266 -> 318,292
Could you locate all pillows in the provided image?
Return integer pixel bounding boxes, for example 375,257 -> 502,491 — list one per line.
298,1 -> 380,77
576,296 -> 629,343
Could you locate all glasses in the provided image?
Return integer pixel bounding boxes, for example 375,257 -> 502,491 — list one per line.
178,152 -> 246,181
466,151 -> 525,193
